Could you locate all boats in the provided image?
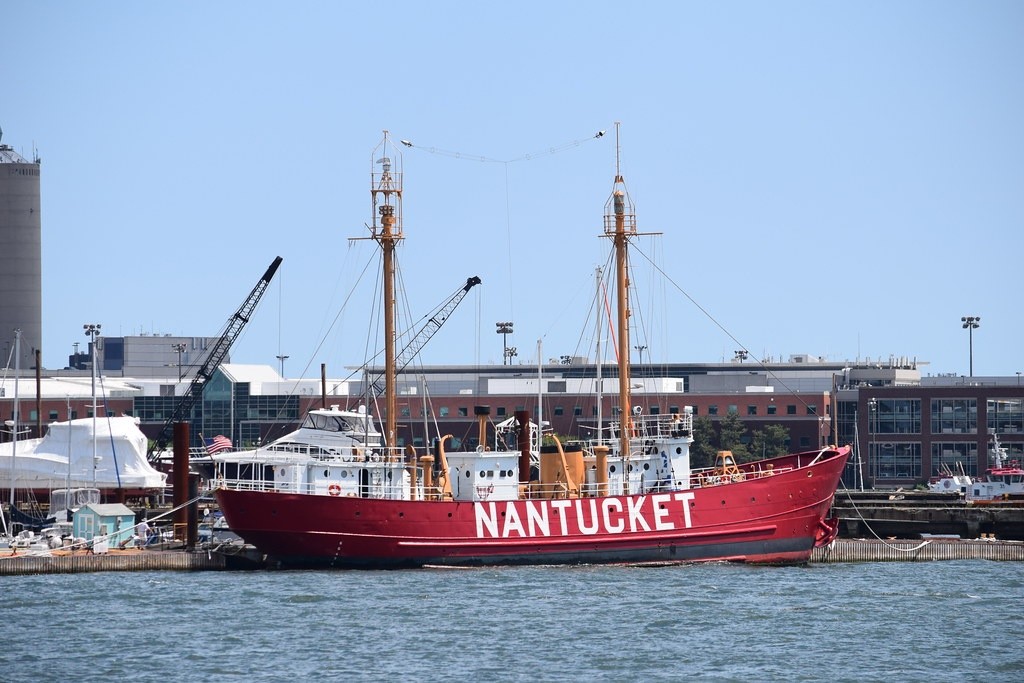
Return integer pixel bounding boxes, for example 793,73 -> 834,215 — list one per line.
189,405 -> 398,489
210,120 -> 851,570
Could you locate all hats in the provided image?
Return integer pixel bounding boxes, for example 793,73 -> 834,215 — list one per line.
141,518 -> 147,521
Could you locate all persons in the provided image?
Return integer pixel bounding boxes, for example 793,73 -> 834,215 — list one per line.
138,518 -> 152,550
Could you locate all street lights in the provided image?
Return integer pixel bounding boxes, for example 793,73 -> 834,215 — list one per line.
497,321 -> 517,365
172,341 -> 187,382
735,350 -> 748,363
83,324 -> 100,398
635,345 -> 647,364
961,316 -> 980,377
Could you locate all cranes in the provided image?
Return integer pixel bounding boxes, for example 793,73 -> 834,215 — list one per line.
346,276 -> 482,412
146,255 -> 284,466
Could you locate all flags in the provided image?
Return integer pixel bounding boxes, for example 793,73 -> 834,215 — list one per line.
204,435 -> 233,453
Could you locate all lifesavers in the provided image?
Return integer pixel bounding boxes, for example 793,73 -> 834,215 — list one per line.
329,484 -> 341,497
476,445 -> 485,455
